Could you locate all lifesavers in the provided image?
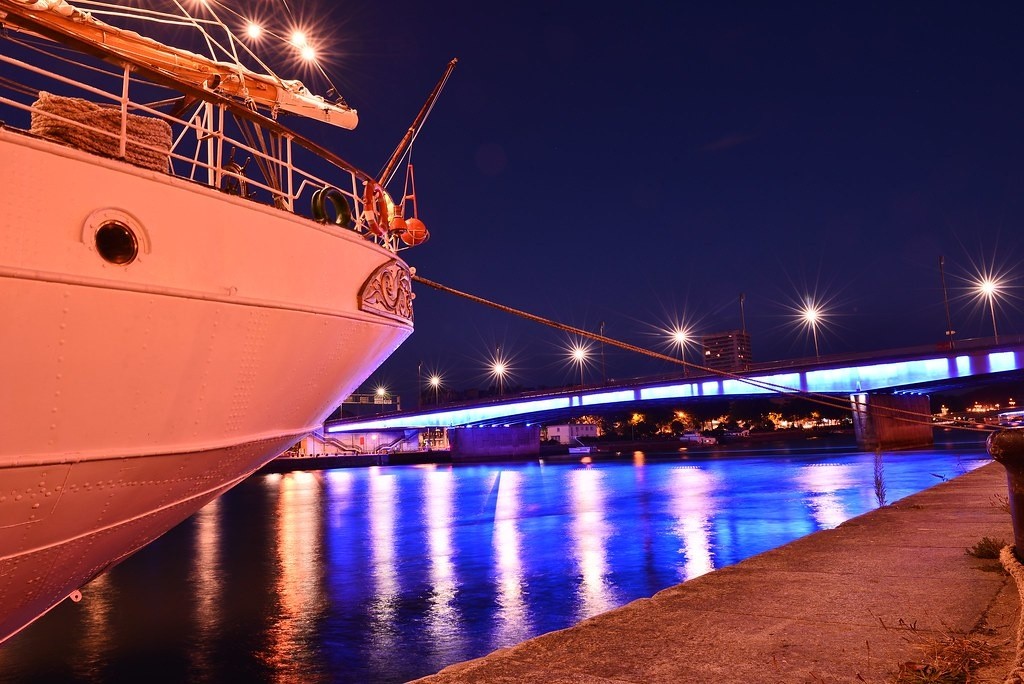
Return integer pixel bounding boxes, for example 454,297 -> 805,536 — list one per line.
310,187 -> 350,225
362,183 -> 389,236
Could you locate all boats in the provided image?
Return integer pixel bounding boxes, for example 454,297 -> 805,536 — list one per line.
0,0 -> 459,648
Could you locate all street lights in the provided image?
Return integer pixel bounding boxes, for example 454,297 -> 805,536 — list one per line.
433,378 -> 438,405
981,281 -> 999,345
808,310 -> 819,357
576,350 -> 584,386
497,365 -> 504,397
379,389 -> 384,412
677,332 -> 686,374
